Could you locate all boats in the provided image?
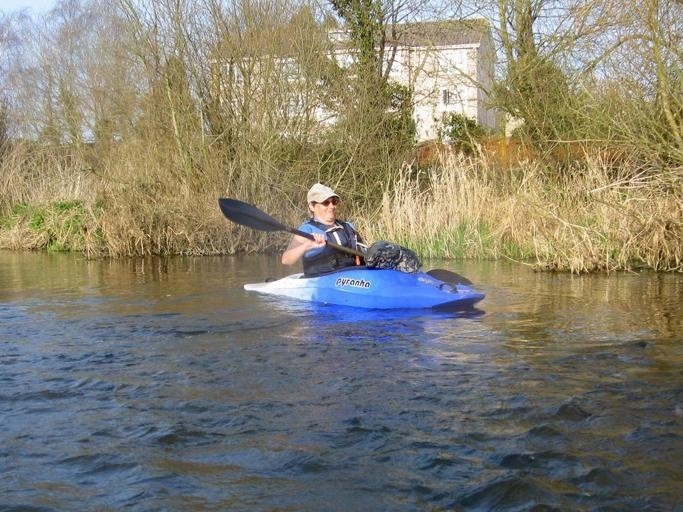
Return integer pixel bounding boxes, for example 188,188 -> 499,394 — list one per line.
244,269 -> 486,310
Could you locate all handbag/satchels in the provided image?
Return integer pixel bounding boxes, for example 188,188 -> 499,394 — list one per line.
364,241 -> 421,274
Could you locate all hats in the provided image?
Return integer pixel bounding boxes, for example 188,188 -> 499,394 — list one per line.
306,183 -> 341,204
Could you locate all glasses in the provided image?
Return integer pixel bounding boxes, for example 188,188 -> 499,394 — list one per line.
322,197 -> 341,206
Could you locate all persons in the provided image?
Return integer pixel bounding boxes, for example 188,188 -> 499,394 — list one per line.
282,183 -> 368,278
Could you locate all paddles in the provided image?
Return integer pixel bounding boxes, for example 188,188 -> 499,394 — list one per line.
218,198 -> 473,285
